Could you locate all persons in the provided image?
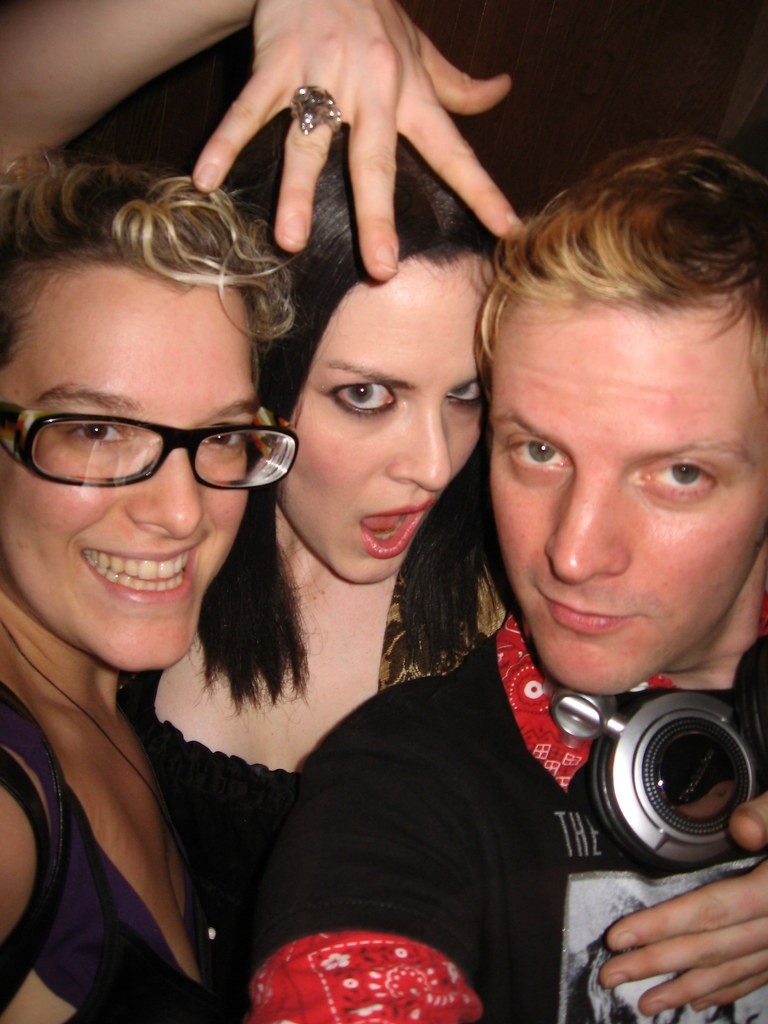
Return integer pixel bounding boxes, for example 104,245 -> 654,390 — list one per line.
2,0 -> 527,1024
1,156 -> 302,1024
240,140 -> 768,1024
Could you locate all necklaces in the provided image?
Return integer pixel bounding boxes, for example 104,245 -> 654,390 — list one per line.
1,612 -> 218,945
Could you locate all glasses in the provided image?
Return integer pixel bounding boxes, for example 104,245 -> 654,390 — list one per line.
0,400 -> 298,490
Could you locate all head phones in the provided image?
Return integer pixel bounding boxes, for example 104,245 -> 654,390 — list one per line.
548,690 -> 763,878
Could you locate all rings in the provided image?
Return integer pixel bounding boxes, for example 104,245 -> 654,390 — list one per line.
290,86 -> 343,136
